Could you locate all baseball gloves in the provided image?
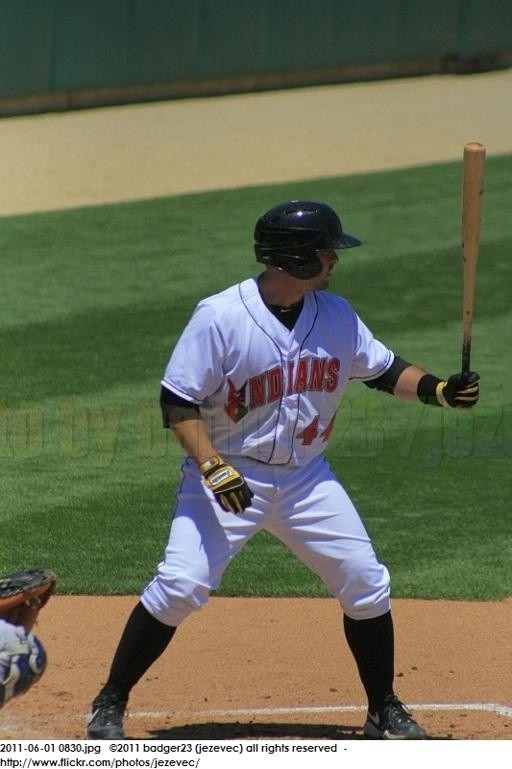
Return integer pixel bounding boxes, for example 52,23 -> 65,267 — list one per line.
1,566 -> 58,635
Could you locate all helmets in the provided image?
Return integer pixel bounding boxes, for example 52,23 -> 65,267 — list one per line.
252,200 -> 362,279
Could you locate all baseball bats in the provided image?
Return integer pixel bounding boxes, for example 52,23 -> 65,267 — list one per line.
462,142 -> 485,372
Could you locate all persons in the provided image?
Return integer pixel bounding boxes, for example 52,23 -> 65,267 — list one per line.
88,202 -> 479,738
0,565 -> 58,707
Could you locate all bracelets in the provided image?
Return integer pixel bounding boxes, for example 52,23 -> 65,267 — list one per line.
418,368 -> 444,409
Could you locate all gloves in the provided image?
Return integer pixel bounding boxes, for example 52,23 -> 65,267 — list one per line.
436,371 -> 479,410
198,455 -> 255,515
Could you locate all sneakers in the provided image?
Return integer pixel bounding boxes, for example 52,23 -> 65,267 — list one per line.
364,693 -> 426,739
87,693 -> 129,740
